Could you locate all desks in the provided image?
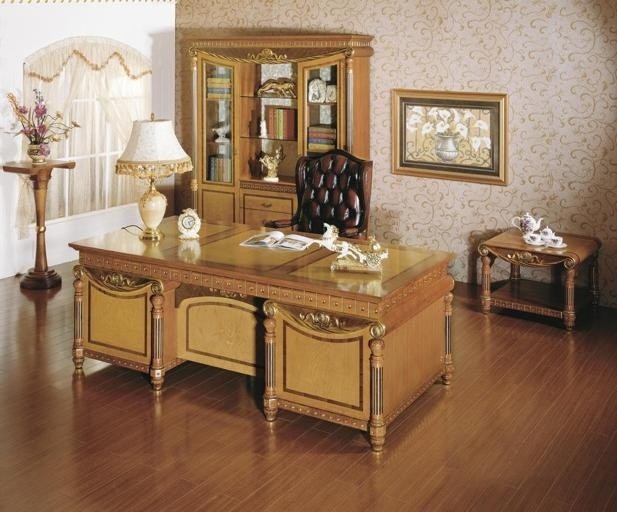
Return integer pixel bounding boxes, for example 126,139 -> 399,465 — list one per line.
63,213 -> 459,457
1,158 -> 78,292
474,220 -> 602,339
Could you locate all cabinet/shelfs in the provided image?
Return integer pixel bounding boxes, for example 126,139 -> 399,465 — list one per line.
181,31 -> 376,232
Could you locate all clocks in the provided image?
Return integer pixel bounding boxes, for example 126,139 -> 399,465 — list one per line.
174,204 -> 204,243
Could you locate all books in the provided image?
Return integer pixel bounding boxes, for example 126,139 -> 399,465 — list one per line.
264,104 -> 297,140
308,126 -> 337,153
239,230 -> 315,252
207,77 -> 232,99
209,154 -> 232,183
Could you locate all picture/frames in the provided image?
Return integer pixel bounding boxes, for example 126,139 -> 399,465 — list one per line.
390,87 -> 510,188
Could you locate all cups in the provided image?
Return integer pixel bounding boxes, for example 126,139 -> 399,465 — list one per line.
523,226 -> 567,249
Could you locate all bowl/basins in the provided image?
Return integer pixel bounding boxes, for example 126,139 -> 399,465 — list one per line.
248,160 -> 266,178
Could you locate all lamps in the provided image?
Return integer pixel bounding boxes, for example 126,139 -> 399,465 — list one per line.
110,108 -> 195,247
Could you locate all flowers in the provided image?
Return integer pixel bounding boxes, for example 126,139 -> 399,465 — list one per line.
0,77 -> 82,146
403,105 -> 492,153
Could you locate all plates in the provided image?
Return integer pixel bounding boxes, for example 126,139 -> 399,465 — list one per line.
308,78 -> 325,103
326,86 -> 336,103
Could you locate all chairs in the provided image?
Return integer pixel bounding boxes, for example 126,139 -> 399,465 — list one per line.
258,146 -> 374,240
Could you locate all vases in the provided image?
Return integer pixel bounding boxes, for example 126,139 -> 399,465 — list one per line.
432,135 -> 460,163
24,142 -> 52,166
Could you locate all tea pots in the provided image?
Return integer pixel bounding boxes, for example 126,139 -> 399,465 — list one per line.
511,212 -> 543,236
